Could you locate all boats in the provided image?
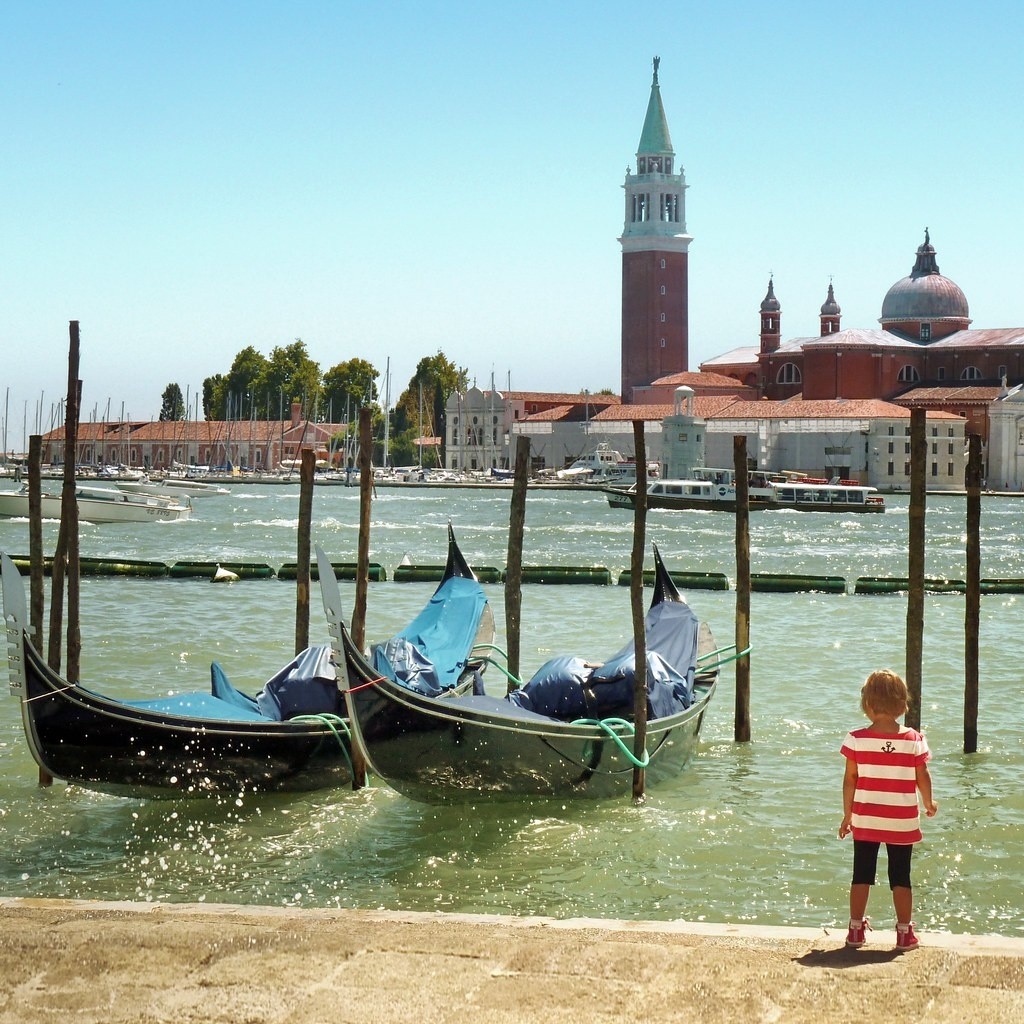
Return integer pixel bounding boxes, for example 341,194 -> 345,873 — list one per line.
0,518 -> 496,800
314,543 -> 721,806
602,467 -> 885,513
114,479 -> 231,497
0,481 -> 193,523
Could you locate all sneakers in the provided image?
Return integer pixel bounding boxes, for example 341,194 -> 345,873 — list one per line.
895,921 -> 920,951
845,916 -> 871,945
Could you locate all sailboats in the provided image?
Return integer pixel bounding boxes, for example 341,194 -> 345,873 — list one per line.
0,357 -> 660,491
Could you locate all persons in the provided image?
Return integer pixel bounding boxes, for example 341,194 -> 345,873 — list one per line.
838,670 -> 939,951
